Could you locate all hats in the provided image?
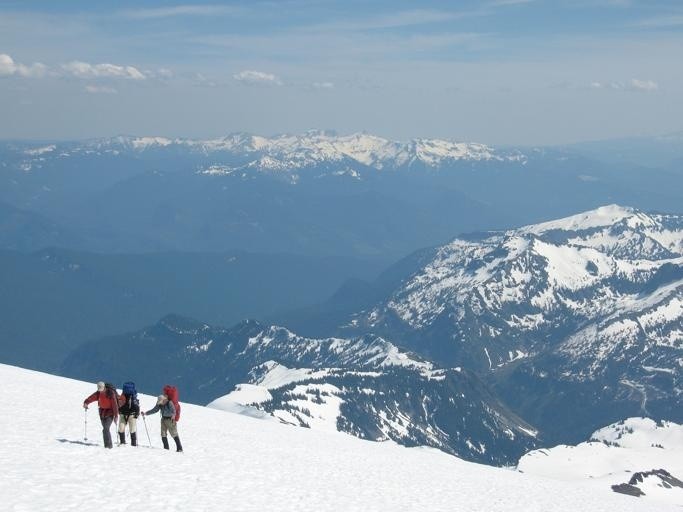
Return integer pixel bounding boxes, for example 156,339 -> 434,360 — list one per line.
97,381 -> 105,392
156,394 -> 167,405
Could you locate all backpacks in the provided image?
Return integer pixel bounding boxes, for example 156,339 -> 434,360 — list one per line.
163,384 -> 181,422
104,382 -> 122,406
122,381 -> 140,405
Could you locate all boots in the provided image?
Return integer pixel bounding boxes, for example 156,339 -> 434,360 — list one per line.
129,432 -> 137,446
161,436 -> 169,449
117,431 -> 126,447
173,435 -> 183,452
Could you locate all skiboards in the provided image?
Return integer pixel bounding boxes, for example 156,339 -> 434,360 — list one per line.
55,439 -> 112,448
116,441 -> 164,450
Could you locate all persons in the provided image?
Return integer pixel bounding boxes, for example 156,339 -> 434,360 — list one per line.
141,395 -> 182,452
83,381 -> 140,449
